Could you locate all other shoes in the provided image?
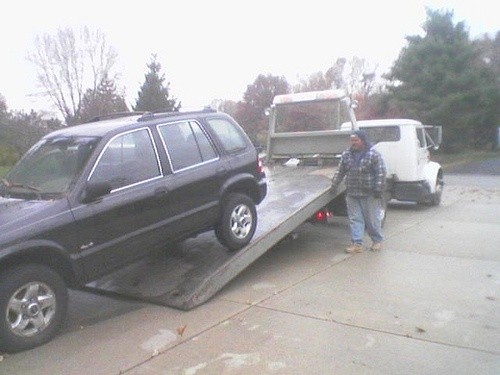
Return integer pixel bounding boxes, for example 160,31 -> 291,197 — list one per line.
370,241 -> 383,251
343,244 -> 366,253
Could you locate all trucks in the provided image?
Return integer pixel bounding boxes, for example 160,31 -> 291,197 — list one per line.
82,90 -> 444,309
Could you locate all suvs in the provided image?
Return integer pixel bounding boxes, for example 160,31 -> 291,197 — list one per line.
0,106 -> 267,348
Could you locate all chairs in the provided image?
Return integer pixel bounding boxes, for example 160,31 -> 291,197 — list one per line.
94,147 -> 127,188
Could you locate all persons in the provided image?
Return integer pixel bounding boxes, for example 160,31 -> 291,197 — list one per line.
331,130 -> 387,253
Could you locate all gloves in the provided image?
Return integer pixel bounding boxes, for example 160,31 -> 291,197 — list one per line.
374,192 -> 381,199
331,187 -> 337,194
